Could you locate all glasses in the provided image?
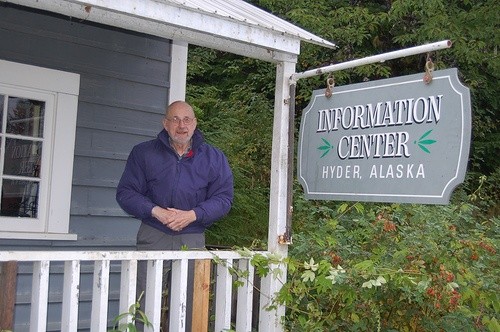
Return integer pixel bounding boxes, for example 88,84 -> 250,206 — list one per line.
165,116 -> 196,124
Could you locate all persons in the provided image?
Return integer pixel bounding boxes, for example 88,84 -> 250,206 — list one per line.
115,100 -> 235,332
